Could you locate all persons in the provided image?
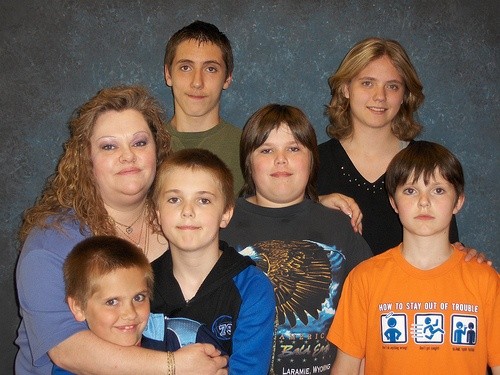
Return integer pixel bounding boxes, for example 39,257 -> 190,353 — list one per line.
218,104 -> 373,375
140,147 -> 276,374
13,85 -> 364,375
327,140 -> 500,375
317,38 -> 493,266
52,234 -> 155,375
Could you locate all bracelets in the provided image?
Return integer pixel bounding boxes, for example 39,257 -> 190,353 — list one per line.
165,350 -> 177,375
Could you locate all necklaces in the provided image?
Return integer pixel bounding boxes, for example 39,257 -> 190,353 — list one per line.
160,18 -> 248,200
111,207 -> 149,257
